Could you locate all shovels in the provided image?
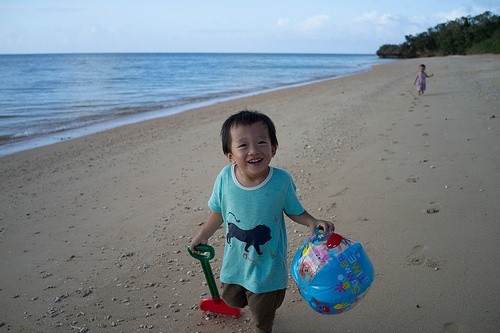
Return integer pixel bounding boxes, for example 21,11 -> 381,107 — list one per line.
186,245 -> 241,316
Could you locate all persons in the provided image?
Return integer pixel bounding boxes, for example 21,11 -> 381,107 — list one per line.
190,111 -> 335,333
412,63 -> 434,97
